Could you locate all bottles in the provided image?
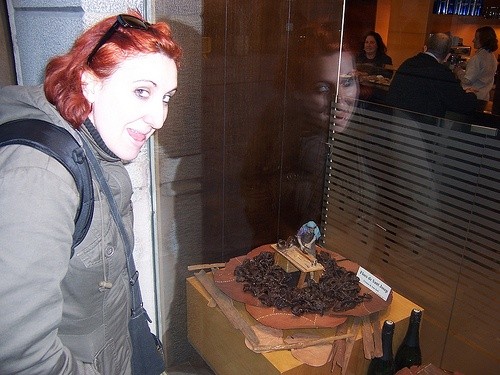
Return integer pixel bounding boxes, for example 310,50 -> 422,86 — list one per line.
367,309 -> 422,375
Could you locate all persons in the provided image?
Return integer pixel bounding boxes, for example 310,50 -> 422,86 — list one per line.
388,30 -> 478,126
0,7 -> 182,375
242,13 -> 439,322
354,31 -> 394,82
459,24 -> 499,112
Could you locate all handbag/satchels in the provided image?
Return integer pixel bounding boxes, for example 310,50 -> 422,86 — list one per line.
129,302 -> 166,375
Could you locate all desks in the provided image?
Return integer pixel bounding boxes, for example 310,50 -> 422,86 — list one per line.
186,268 -> 425,375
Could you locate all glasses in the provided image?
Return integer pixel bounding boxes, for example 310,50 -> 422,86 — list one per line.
290,20 -> 342,47
86,14 -> 154,62
